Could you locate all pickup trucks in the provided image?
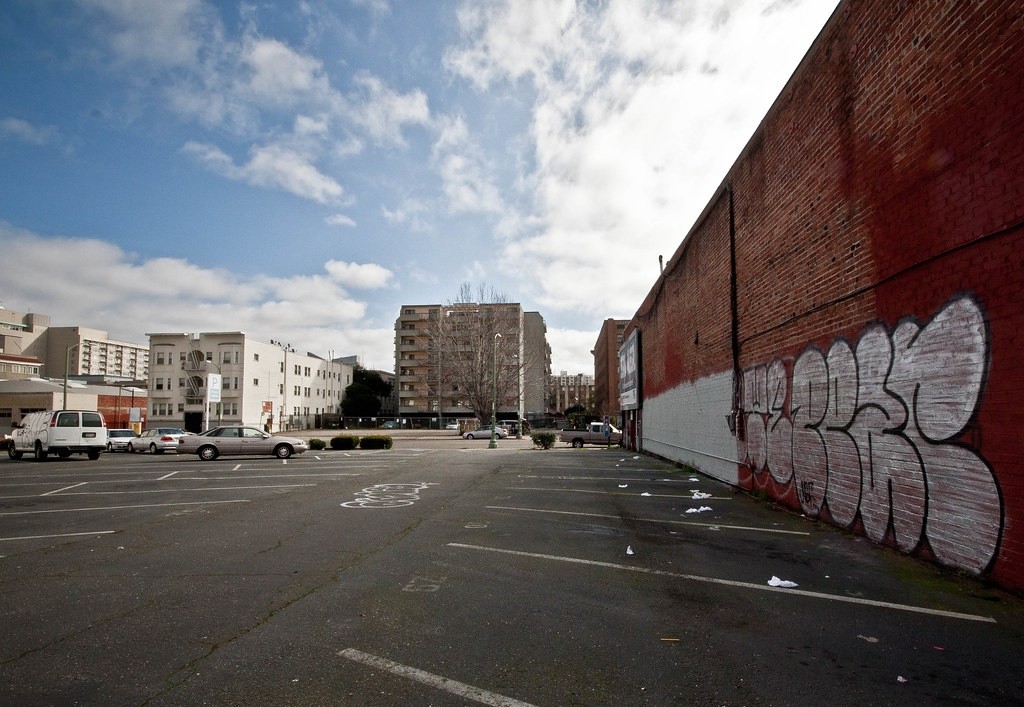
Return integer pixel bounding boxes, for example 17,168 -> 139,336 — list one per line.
559,422 -> 622,448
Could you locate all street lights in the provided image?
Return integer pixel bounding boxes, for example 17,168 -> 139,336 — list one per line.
118,384 -> 125,429
205,360 -> 221,426
63,342 -> 98,410
488,333 -> 502,448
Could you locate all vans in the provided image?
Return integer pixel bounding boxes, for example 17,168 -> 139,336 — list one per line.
8,409 -> 108,462
490,420 -> 519,435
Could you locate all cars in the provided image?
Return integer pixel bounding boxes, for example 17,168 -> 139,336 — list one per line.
107,428 -> 139,453
446,421 -> 461,430
127,427 -> 195,454
381,420 -> 400,429
176,426 -> 309,461
463,424 -> 508,440
